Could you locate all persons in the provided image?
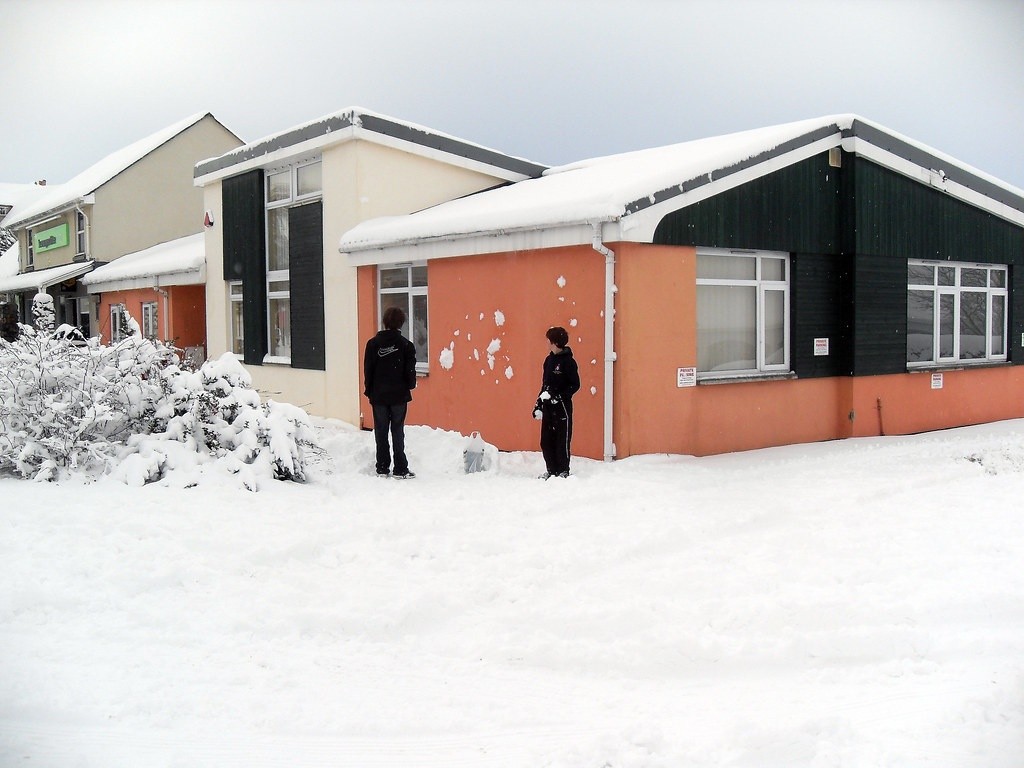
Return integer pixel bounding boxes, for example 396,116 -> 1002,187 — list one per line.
364,307 -> 418,479
532,325 -> 581,481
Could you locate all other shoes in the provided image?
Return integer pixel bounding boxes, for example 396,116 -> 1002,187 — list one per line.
538,471 -> 552,481
392,468 -> 415,479
556,471 -> 568,479
376,462 -> 390,478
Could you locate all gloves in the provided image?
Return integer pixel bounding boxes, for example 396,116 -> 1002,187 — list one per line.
537,398 -> 551,407
532,406 -> 543,420
